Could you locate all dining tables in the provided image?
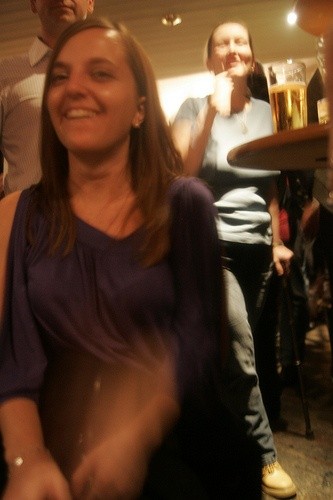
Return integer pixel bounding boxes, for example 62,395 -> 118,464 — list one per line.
227,121 -> 333,170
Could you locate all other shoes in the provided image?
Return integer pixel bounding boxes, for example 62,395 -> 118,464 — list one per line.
259,461 -> 297,500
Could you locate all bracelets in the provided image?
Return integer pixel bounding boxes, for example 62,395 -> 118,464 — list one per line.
8,446 -> 48,476
271,240 -> 284,246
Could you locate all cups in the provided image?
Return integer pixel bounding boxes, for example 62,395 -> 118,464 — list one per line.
267,60 -> 307,134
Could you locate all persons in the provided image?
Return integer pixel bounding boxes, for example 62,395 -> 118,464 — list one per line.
169,21 -> 298,498
0,16 -> 265,500
0,0 -> 94,198
